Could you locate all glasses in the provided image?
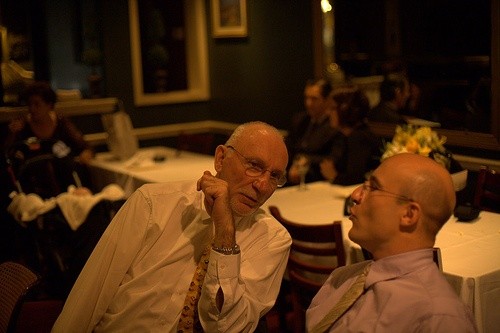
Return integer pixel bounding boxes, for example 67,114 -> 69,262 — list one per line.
365,176 -> 414,202
226,145 -> 287,187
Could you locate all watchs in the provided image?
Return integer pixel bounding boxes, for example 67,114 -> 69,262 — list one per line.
211,244 -> 240,254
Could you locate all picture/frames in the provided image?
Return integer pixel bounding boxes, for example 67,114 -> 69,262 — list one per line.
210,0 -> 249,39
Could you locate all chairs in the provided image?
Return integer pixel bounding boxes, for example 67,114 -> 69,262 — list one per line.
473,165 -> 500,213
268,206 -> 345,333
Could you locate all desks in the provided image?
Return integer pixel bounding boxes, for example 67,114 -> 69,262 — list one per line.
72,145 -> 500,333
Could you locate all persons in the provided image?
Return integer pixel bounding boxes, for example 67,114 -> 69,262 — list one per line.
284,69 -> 420,184
305,153 -> 477,333
0,84 -> 95,235
49,120 -> 293,333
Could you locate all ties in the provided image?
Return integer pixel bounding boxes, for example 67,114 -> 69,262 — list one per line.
309,262 -> 370,333
178,236 -> 216,333
